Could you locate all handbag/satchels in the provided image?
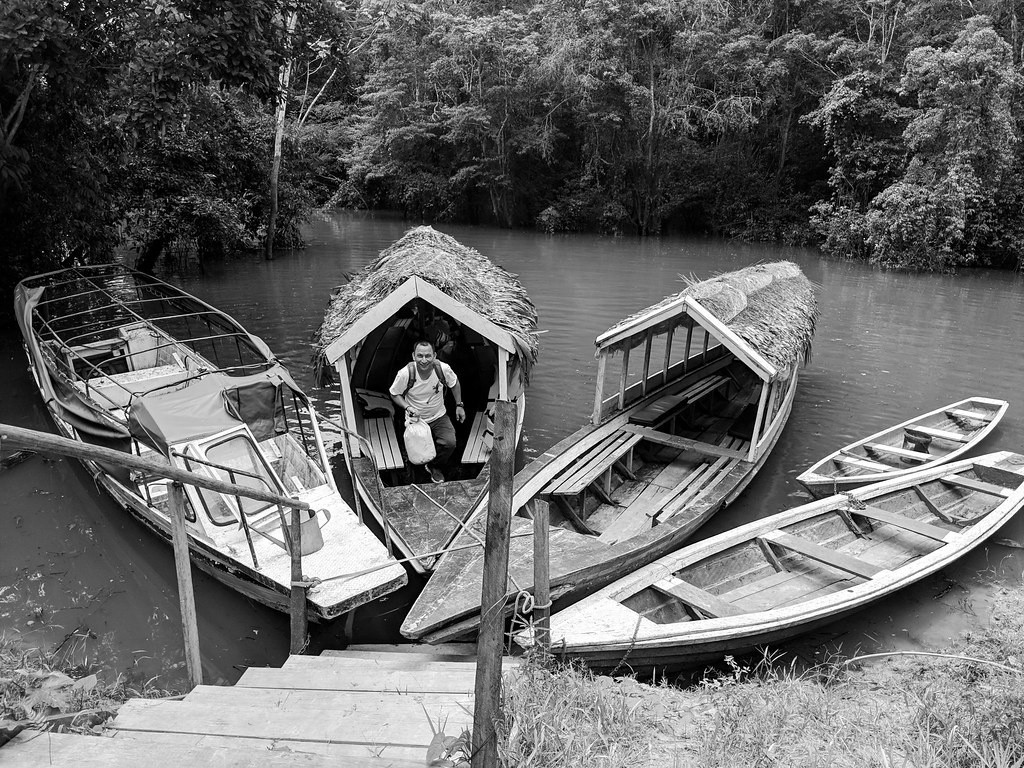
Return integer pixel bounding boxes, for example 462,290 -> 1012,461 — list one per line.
403,410 -> 437,465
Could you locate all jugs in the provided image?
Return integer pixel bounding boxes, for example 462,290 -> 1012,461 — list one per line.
238,508 -> 332,558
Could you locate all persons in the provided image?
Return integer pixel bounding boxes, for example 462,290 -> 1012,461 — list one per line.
389,339 -> 465,483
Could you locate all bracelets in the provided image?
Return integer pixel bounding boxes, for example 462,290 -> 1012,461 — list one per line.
457,402 -> 464,406
405,406 -> 409,410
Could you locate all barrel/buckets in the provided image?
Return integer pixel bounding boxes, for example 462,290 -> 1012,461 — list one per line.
900,430 -> 932,465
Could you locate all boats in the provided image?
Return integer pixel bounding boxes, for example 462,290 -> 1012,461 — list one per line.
796,395 -> 1009,499
321,226 -> 540,573
12,259 -> 407,622
513,450 -> 1024,677
401,259 -> 820,643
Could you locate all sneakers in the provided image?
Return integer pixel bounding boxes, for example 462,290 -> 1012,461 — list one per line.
425,462 -> 445,484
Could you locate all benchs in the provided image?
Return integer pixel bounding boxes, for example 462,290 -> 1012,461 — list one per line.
758,524 -> 894,582
864,433 -> 942,462
540,372 -> 731,498
832,453 -> 903,472
655,576 -> 746,617
646,386 -> 763,523
944,472 -> 1015,500
71,364 -> 189,411
840,502 -> 960,546
945,407 -> 994,423
361,318 -> 413,473
905,424 -> 974,443
460,326 -> 500,467
